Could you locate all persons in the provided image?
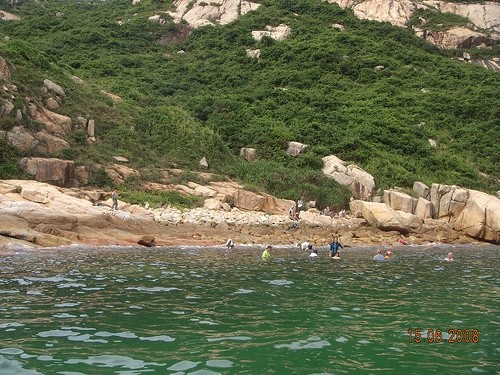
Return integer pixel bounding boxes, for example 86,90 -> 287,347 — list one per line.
305,244 -> 312,251
399,238 -> 404,244
329,236 -> 343,257
384,251 -> 390,258
323,207 -> 330,214
448,252 -> 454,261
374,250 -> 384,261
112,188 -> 118,210
262,246 -> 272,261
290,206 -> 294,220
310,250 -> 318,256
226,238 -> 234,250
298,197 -> 303,212
